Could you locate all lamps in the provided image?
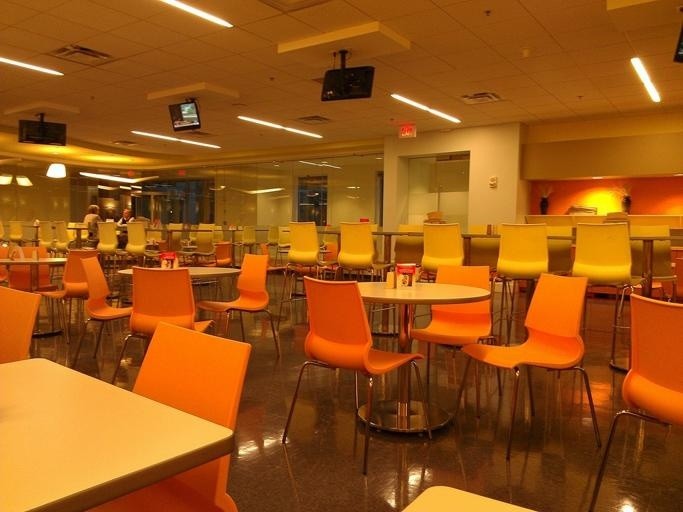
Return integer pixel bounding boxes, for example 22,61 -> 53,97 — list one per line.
46,163 -> 66,179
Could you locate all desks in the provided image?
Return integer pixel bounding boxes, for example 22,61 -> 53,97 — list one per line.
402,485 -> 533,512
0,257 -> 68,339
1,357 -> 235,512
118,266 -> 241,278
547,236 -> 576,241
371,232 -> 423,280
461,234 -> 500,266
358,281 -> 490,436
319,231 -> 341,256
631,236 -> 683,299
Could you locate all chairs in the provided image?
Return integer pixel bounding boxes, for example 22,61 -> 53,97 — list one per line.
395,224 -> 424,266
9,246 -> 55,293
37,220 -> 53,248
449,272 -> 601,461
239,226 -> 256,261
492,222 -> 568,311
414,263 -> 501,418
275,226 -> 292,266
54,221 -> 74,253
39,250 -> 99,343
545,224 -> 572,276
281,221 -> 337,302
111,266 -> 214,386
125,221 -> 162,268
338,222 -> 393,281
571,223 -> 647,352
613,225 -> 677,326
0,220 -> 35,242
264,226 -> 277,248
73,256 -> 132,364
86,322 -> 251,512
0,286 -> 40,364
182,224 -> 215,266
468,224 -> 501,272
282,275 -> 433,474
324,226 -> 340,244
586,293 -> 683,512
96,221 -> 126,273
368,224 -> 376,251
421,223 -> 464,282
197,253 -> 280,359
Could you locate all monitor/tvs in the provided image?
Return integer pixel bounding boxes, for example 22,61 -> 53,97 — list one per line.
321,65 -> 376,100
19,117 -> 69,147
168,101 -> 201,131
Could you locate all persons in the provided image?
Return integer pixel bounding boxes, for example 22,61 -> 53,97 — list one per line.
116,208 -> 137,229
82,205 -> 104,236
